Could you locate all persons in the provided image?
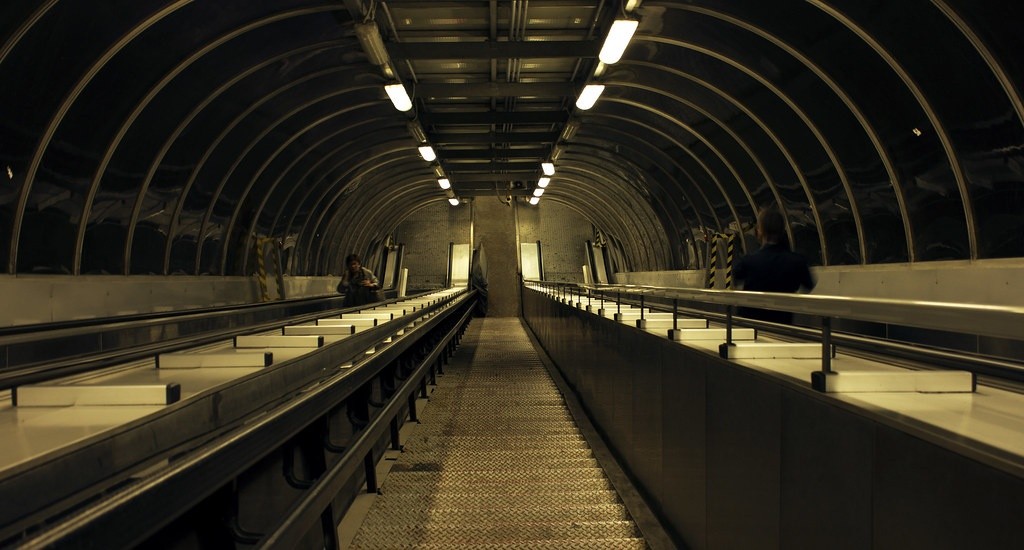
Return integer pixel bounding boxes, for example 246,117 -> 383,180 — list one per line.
735,209 -> 814,328
337,253 -> 380,309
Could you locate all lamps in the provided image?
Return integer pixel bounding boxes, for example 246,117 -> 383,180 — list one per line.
353,9 -> 642,209
